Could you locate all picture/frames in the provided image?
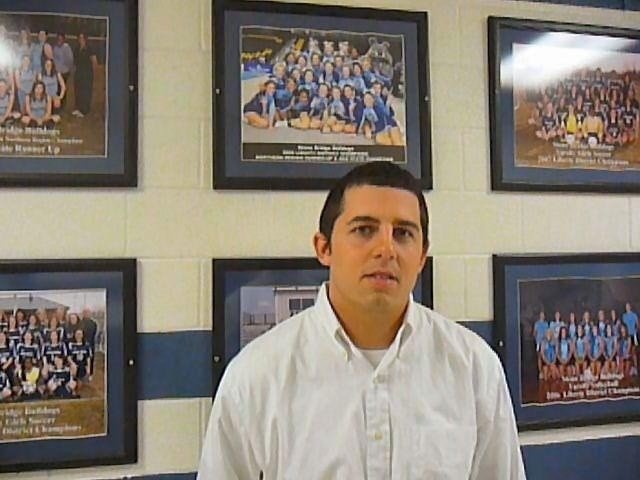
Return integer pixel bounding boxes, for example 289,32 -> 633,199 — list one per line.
0,0 -> 139,188
487,14 -> 639,193
0,257 -> 139,474
492,251 -> 640,430
210,0 -> 434,190
211,256 -> 434,403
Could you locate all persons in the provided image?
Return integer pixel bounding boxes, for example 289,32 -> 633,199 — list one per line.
1,15 -> 107,134
533,302 -> 639,383
195,161 -> 529,480
242,35 -> 405,148
0,302 -> 97,399
527,67 -> 640,145
